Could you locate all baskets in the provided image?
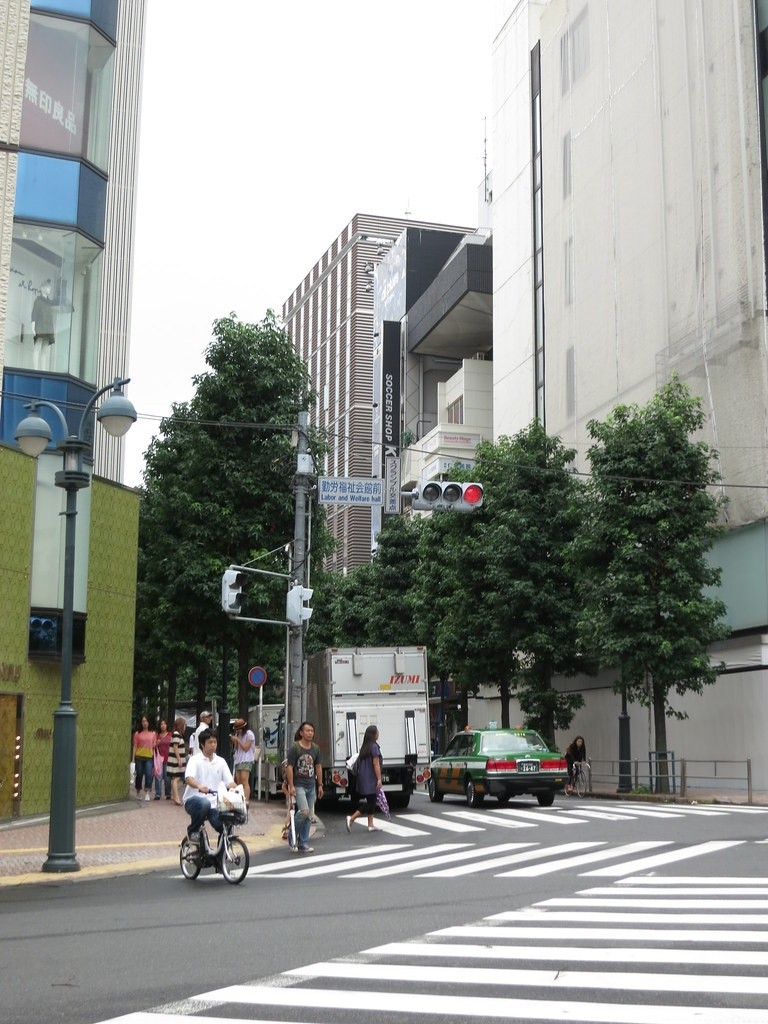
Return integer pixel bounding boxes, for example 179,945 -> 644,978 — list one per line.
217,801 -> 249,823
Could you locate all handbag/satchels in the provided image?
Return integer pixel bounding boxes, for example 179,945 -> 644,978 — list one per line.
153,747 -> 164,777
130,761 -> 137,783
218,781 -> 247,815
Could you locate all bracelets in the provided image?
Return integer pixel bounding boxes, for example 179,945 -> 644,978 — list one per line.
319,783 -> 323,786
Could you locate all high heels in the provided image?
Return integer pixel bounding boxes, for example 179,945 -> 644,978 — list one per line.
172,798 -> 183,805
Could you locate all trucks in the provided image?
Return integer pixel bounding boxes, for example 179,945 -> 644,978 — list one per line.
264,647 -> 436,809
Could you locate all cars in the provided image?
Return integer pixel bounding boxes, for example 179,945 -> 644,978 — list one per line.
427,727 -> 571,808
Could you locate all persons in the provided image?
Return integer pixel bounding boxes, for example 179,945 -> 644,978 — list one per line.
346,725 -> 384,833
31,282 -> 55,371
230,719 -> 255,810
166,718 -> 187,806
565,736 -> 586,791
188,733 -> 195,760
154,719 -> 173,800
182,728 -> 238,874
131,714 -> 160,801
193,711 -> 212,755
282,722 -> 323,852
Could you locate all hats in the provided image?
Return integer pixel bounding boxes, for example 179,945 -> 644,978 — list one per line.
200,711 -> 213,719
233,719 -> 247,729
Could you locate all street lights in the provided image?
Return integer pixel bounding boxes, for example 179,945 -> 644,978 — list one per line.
14,378 -> 139,875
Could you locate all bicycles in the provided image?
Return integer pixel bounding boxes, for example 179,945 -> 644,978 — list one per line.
565,762 -> 592,800
178,787 -> 252,885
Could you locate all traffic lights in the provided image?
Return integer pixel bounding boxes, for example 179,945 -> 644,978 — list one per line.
413,481 -> 486,513
286,585 -> 315,626
221,570 -> 249,613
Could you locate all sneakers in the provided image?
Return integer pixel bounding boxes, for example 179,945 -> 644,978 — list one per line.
187,828 -> 201,844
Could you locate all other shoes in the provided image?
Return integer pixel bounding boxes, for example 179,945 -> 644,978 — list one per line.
368,826 -> 382,831
288,838 -> 298,852
298,844 -> 314,853
166,797 -> 171,800
137,792 -> 142,800
568,784 -> 573,791
145,794 -> 150,801
346,815 -> 351,832
154,797 -> 160,800
